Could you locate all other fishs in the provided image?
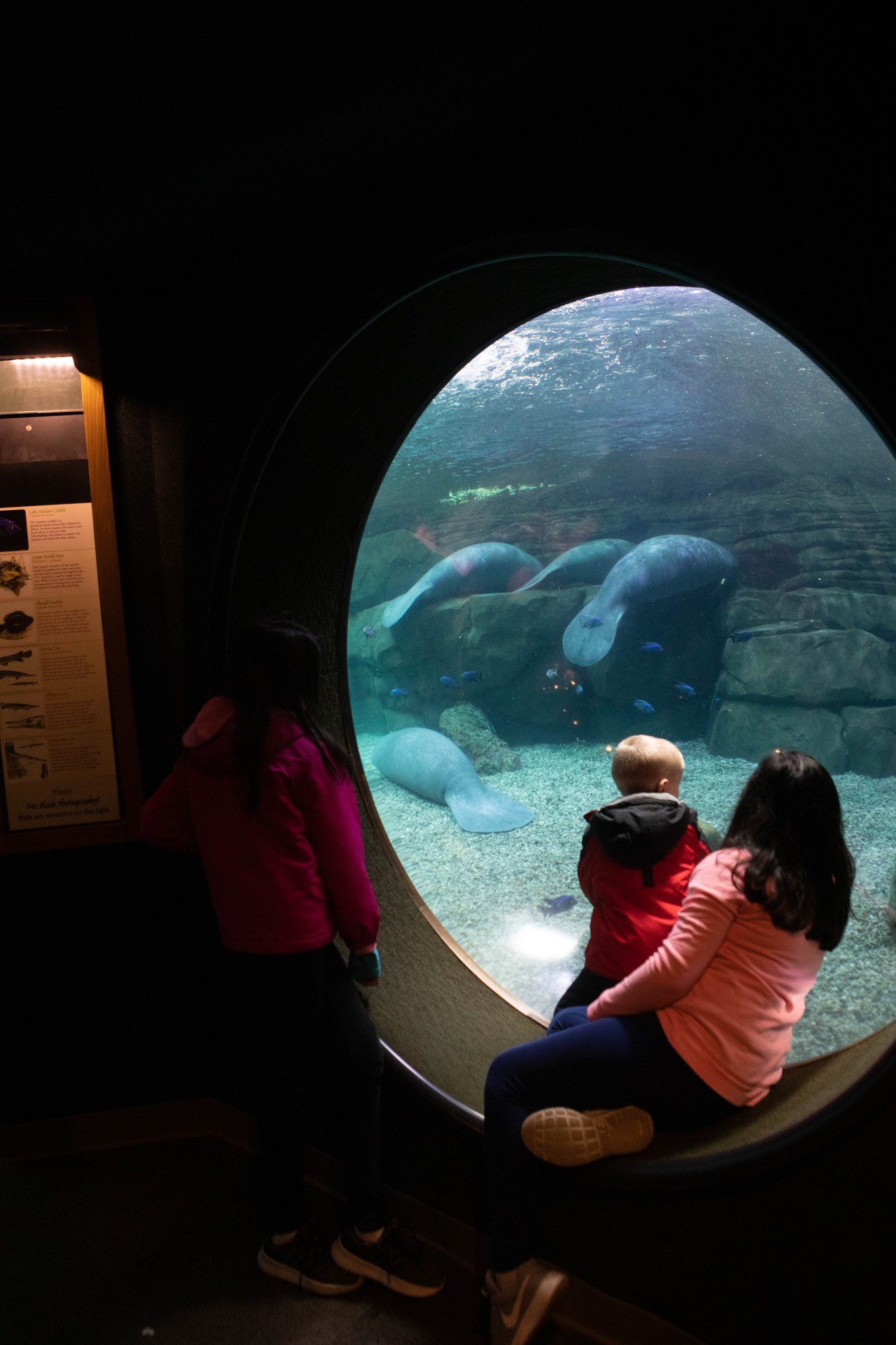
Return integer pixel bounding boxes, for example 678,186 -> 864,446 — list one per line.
507,538 -> 646,591
379,540 -> 543,629
370,726 -> 533,834
561,534 -> 741,665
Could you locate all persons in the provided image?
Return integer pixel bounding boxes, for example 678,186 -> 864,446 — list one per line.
555,734 -> 712,1014
482,751 -> 856,1345
142,618 -> 449,1296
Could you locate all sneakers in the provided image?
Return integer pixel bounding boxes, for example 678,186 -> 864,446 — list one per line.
484,1260 -> 568,1345
258,1233 -> 363,1295
331,1217 -> 447,1297
522,1106 -> 654,1166
